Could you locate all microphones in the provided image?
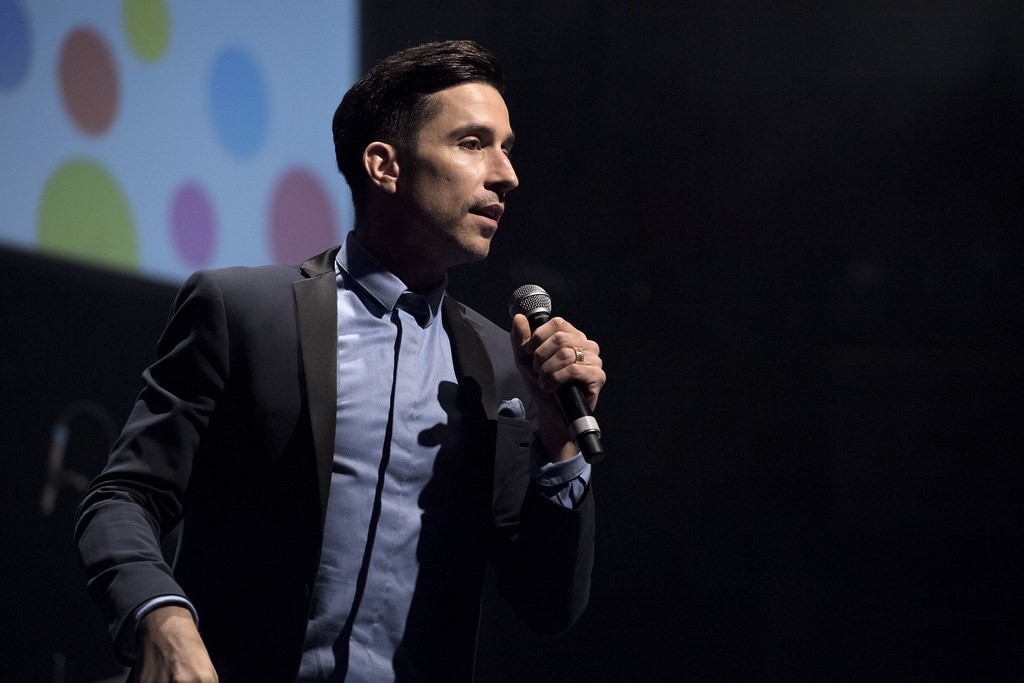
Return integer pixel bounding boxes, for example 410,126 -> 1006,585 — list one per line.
510,285 -> 605,464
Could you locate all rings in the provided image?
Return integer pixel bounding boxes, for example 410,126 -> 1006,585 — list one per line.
572,346 -> 585,364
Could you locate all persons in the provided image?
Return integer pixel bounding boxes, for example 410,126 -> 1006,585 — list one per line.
74,40 -> 607,683
